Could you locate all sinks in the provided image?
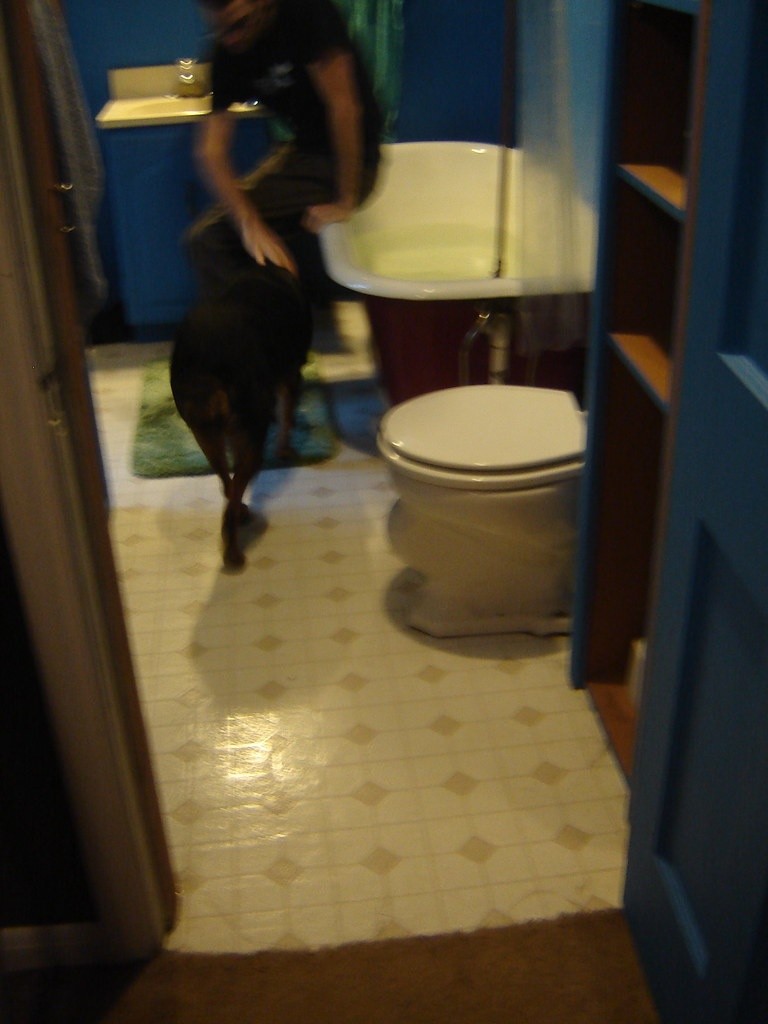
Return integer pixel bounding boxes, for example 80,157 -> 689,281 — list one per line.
95,96 -> 212,129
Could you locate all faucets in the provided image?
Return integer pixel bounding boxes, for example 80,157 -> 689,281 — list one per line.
176,58 -> 197,97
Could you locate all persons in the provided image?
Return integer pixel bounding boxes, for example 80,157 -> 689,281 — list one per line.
185,2 -> 379,276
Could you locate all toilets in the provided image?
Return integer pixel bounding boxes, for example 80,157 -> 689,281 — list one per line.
376,384 -> 587,638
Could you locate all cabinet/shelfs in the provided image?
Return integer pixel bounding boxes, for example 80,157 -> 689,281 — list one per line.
570,1 -> 705,789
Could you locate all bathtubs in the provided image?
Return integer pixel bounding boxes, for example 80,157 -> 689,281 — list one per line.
316,141 -> 598,407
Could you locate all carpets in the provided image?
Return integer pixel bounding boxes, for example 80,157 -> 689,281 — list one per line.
133,351 -> 340,479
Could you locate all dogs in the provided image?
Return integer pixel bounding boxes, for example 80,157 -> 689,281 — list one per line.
169,220 -> 320,571
